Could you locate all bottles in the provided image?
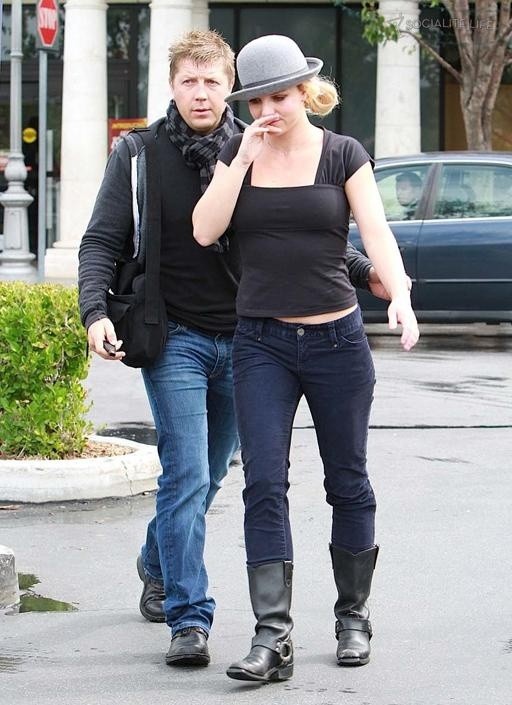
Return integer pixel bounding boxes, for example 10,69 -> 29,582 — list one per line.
221,30 -> 326,106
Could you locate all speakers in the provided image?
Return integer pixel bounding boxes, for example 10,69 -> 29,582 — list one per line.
226,558 -> 296,687
325,540 -> 381,668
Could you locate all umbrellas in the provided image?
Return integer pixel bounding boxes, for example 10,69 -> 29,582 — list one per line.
134,550 -> 168,625
161,626 -> 213,673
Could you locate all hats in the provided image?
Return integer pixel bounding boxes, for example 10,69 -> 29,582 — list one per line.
347,150 -> 512,328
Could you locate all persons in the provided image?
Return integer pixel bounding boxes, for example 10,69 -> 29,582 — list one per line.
384,172 -> 424,221
74,25 -> 412,667
192,32 -> 424,688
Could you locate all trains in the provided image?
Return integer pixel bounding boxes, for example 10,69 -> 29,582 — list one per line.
37,0 -> 58,47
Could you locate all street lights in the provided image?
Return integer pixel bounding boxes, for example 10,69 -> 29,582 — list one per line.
107,126 -> 168,372
